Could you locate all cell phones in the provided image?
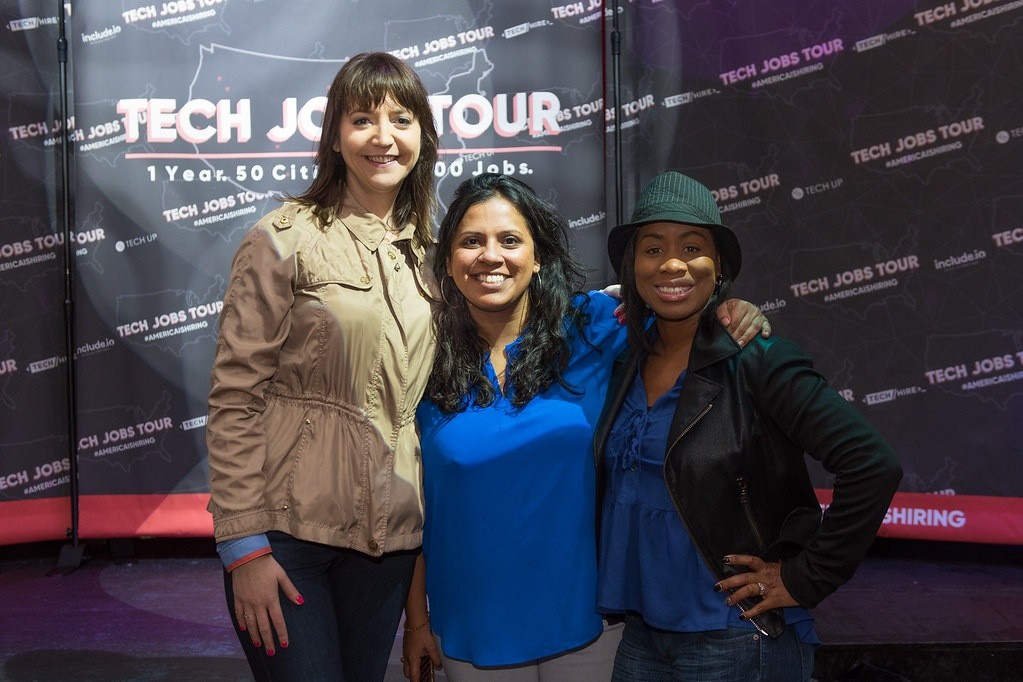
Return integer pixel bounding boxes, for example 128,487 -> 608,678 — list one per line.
419,656 -> 435,682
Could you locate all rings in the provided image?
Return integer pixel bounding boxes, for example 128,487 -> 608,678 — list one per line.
242,611 -> 256,620
400,656 -> 407,664
757,582 -> 766,597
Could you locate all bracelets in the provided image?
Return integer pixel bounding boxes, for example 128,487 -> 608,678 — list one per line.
403,612 -> 430,632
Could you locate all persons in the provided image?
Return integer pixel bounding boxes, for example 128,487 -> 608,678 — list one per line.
203,51 -> 902,682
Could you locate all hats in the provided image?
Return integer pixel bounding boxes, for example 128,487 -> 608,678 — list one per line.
608,172 -> 741,281
